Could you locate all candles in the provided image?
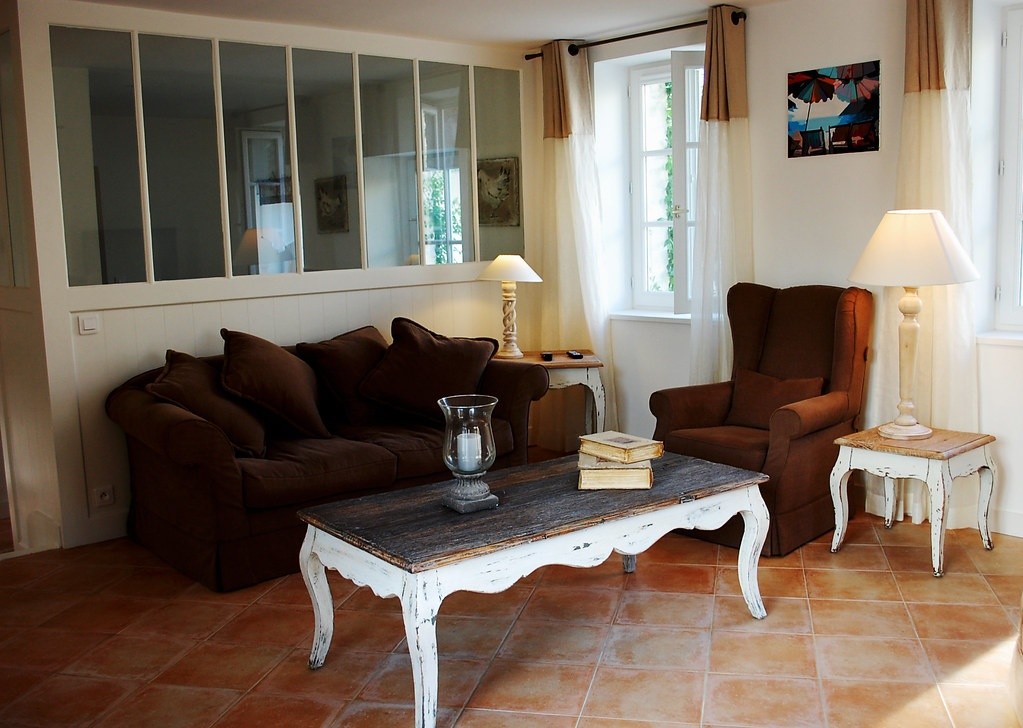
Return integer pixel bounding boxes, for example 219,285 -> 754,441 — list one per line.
457,431 -> 481,470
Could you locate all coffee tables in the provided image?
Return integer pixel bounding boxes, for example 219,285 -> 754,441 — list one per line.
297,452 -> 772,728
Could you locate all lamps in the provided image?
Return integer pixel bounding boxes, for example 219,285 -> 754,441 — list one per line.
847,210 -> 981,441
474,250 -> 546,359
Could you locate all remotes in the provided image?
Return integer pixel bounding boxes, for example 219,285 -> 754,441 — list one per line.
565,350 -> 583,359
540,351 -> 554,361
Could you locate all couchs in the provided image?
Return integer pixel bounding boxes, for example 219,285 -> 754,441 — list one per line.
650,279 -> 877,560
110,346 -> 552,594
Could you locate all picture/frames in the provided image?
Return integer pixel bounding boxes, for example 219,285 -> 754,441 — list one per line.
474,155 -> 520,227
313,174 -> 349,235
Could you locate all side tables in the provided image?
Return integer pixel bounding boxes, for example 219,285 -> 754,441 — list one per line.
830,425 -> 1000,577
491,348 -> 605,434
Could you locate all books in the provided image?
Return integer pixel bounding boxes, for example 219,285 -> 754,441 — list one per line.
578,431 -> 665,490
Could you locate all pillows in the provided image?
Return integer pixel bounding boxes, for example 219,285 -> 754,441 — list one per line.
726,365 -> 823,433
145,347 -> 264,454
295,325 -> 386,421
380,318 -> 499,419
218,325 -> 332,439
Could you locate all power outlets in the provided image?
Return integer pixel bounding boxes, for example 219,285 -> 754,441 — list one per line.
93,484 -> 115,509
